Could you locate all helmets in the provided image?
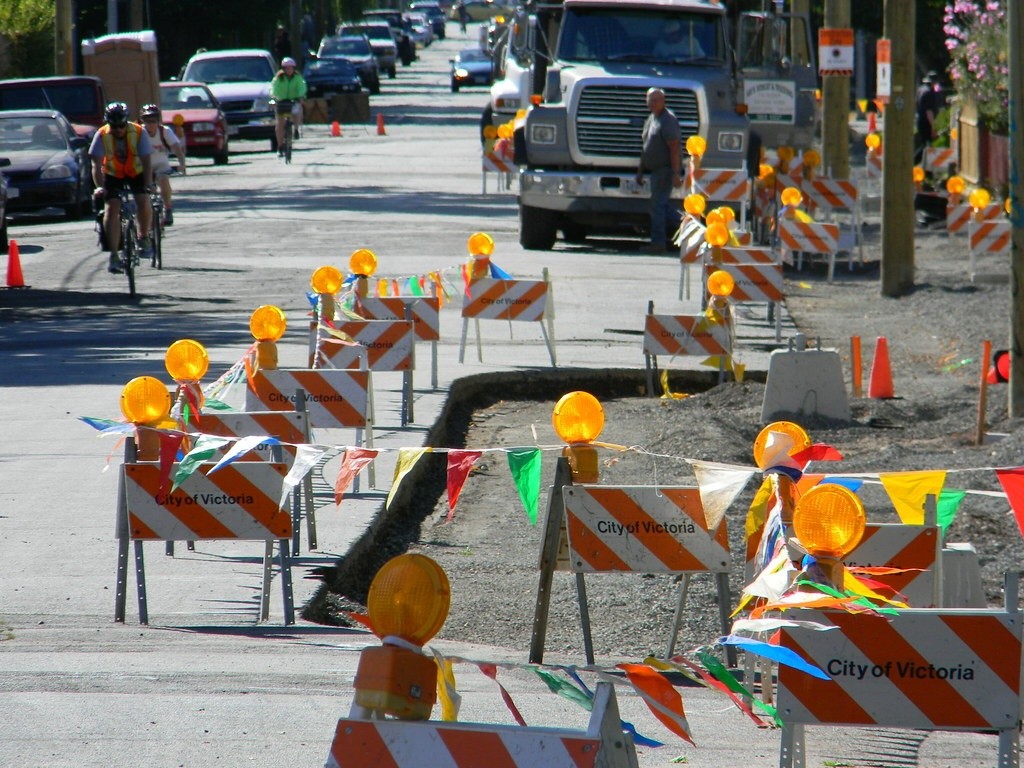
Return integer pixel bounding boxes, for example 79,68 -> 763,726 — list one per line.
281,57 -> 296,67
104,102 -> 130,124
139,105 -> 160,119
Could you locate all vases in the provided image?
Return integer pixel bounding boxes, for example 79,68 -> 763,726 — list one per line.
988,130 -> 1009,194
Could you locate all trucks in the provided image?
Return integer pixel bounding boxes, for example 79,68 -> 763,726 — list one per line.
481,0 -> 818,250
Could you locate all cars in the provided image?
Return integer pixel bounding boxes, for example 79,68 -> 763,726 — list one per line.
159,82 -> 230,164
336,21 -> 397,79
302,57 -> 361,99
0,110 -> 96,219
450,0 -> 513,22
0,157 -> 11,254
362,0 -> 446,66
448,49 -> 495,92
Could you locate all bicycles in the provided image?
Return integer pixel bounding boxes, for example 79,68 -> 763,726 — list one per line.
148,165 -> 178,270
275,101 -> 300,165
109,184 -> 152,296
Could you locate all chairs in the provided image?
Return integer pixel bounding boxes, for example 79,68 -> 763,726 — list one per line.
31,124 -> 52,145
247,66 -> 264,81
188,96 -> 201,105
201,66 -> 216,81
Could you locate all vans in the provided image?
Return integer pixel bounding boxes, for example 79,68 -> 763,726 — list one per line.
178,49 -> 280,153
0,76 -> 105,142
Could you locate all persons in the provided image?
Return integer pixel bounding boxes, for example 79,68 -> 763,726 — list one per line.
87,103 -> 153,273
140,103 -> 185,226
21,125 -> 60,150
643,20 -> 705,64
912,71 -> 939,164
636,88 -> 683,255
458,3 -> 466,33
269,57 -> 307,157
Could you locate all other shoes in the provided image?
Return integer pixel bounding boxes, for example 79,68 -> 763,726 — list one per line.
294,128 -> 300,140
277,148 -> 283,157
640,244 -> 662,256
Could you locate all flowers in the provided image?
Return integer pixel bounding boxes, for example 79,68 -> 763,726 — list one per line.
938,0 -> 1009,136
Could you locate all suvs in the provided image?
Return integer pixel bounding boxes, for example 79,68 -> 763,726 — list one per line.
308,34 -> 381,94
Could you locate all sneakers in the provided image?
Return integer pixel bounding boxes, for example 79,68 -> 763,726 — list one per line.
139,236 -> 153,259
162,207 -> 174,226
108,255 -> 120,272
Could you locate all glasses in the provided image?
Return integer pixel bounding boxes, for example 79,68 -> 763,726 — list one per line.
109,122 -> 127,129
145,120 -> 157,124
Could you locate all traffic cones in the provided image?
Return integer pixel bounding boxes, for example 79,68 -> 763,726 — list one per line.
867,336 -> 904,400
988,350 -> 1010,385
376,113 -> 385,135
5,240 -> 26,288
329,121 -> 343,137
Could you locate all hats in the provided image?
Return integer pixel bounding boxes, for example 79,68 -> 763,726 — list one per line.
921,71 -> 936,83
